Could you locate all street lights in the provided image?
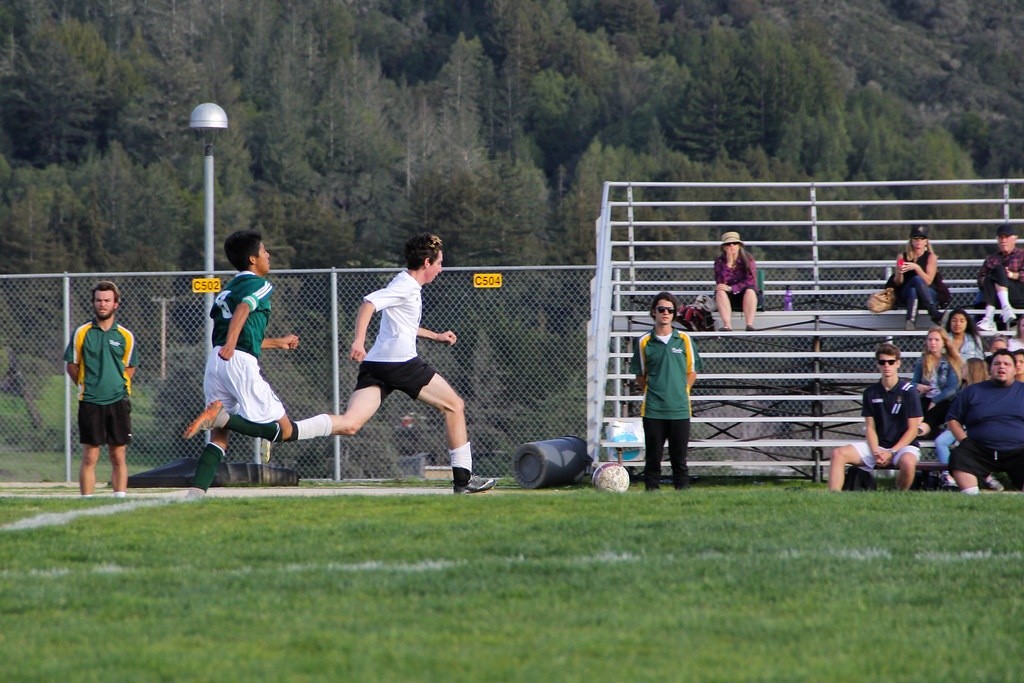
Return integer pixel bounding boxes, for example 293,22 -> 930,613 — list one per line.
188,101 -> 230,449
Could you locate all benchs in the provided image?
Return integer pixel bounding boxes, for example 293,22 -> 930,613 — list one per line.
584,178 -> 1024,486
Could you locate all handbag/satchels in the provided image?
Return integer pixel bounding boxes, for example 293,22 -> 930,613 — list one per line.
675,306 -> 716,330
972,290 -> 988,309
867,287 -> 895,312
842,465 -> 876,491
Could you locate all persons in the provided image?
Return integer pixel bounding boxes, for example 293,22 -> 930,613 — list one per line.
894,225 -> 946,330
628,292 -> 701,491
946,349 -> 1024,493
1007,315 -> 1024,352
182,230 -> 299,497
1013,349 -> 1024,382
286,232 -> 497,494
62,281 -> 138,496
713,232 -> 764,331
977,224 -> 1024,331
829,342 -> 924,491
945,308 -> 984,360
910,326 -> 957,440
933,358 -> 1004,491
984,333 -> 1009,363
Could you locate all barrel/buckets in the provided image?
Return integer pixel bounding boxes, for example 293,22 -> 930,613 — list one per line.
513,436 -> 593,490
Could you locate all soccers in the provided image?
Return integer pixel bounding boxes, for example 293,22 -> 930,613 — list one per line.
593,461 -> 630,493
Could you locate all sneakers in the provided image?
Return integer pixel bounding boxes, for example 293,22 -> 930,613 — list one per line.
184,401 -> 225,438
452,474 -> 497,494
261,438 -> 272,462
976,317 -> 997,331
1000,305 -> 1017,323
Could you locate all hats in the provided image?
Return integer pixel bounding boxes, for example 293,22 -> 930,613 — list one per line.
996,223 -> 1018,235
720,231 -> 743,246
909,226 -> 928,238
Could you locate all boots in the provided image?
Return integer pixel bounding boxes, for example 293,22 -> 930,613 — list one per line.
904,299 -> 918,330
926,304 -> 947,327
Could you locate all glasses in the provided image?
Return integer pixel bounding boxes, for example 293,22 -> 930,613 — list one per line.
653,305 -> 675,313
878,359 -> 897,365
914,236 -> 926,240
724,242 -> 740,246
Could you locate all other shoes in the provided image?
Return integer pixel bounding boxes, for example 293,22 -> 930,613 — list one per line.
985,476 -> 1004,491
745,324 -> 755,331
941,479 -> 958,491
719,323 -> 733,331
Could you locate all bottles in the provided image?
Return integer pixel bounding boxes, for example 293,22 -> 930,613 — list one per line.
784,285 -> 793,311
